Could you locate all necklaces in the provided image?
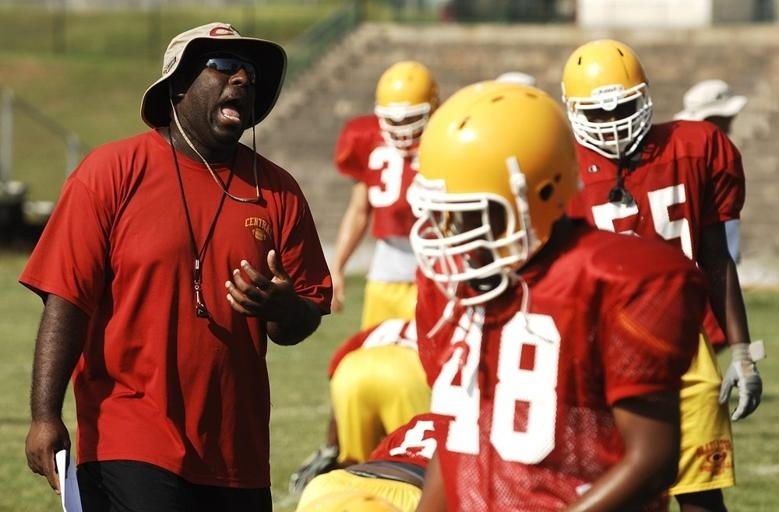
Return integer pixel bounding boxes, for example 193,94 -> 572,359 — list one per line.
164,119 -> 236,314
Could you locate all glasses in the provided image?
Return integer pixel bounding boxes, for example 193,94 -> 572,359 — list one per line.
187,54 -> 259,87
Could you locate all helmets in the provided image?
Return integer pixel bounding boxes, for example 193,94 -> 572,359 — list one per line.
560,39 -> 654,160
374,61 -> 440,160
405,80 -> 583,307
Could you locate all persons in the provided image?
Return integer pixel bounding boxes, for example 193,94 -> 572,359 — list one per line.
334,61 -> 439,329
29,22 -> 332,512
676,78 -> 746,136
562,40 -> 761,512
294,80 -> 693,509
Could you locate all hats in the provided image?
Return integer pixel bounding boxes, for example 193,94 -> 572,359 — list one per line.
673,80 -> 749,121
140,22 -> 288,129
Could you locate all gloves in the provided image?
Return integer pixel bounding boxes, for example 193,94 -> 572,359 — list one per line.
718,340 -> 767,422
